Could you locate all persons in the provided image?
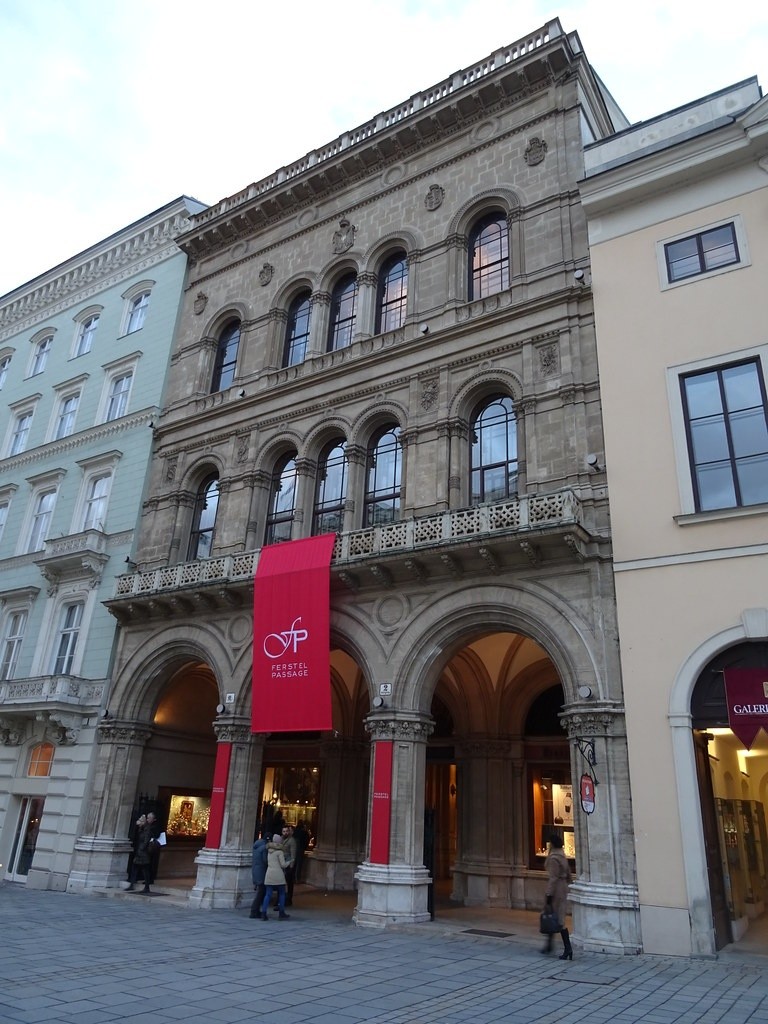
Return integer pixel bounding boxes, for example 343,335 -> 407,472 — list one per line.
250,810 -> 310,921
541,835 -> 573,959
124,812 -> 161,893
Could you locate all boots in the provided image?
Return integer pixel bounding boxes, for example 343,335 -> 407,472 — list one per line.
123,880 -> 136,891
538,933 -> 554,955
139,880 -> 149,893
261,911 -> 268,920
279,911 -> 290,921
559,928 -> 573,959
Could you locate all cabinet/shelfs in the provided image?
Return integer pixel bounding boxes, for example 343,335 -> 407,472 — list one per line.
715,796 -> 768,920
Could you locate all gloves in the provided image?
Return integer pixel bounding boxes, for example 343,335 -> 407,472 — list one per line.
547,896 -> 552,904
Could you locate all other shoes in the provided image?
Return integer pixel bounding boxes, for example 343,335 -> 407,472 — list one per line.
250,910 -> 262,918
273,905 -> 280,911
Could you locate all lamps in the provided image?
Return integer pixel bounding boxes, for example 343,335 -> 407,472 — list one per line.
554,799 -> 564,824
146,421 -> 157,429
578,686 -> 594,698
238,389 -> 246,396
372,697 -> 388,708
101,710 -> 109,718
123,555 -> 137,567
216,704 -> 230,715
574,269 -> 585,285
420,324 -> 429,334
587,454 -> 600,472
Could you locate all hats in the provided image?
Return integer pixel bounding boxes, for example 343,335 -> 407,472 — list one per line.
263,833 -> 272,842
136,815 -> 146,825
273,834 -> 282,844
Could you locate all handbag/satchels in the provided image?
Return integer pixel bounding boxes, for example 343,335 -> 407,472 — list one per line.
156,832 -> 166,846
145,830 -> 159,855
540,903 -> 561,934
